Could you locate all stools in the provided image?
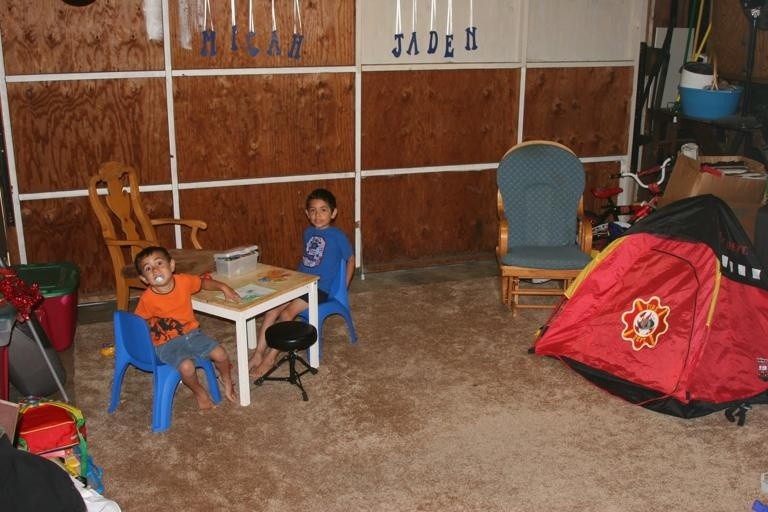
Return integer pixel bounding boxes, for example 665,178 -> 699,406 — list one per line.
255,320 -> 318,403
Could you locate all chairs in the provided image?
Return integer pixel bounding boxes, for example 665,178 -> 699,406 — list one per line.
493,140 -> 602,318
87,159 -> 219,432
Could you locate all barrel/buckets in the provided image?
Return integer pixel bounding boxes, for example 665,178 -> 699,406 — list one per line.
680,62 -> 718,90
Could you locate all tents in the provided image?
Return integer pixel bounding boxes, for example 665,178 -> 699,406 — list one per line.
526,193 -> 768,420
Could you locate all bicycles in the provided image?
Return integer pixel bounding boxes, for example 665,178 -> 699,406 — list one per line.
584,153 -> 677,240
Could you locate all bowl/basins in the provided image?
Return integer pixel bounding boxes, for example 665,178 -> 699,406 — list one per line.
678,84 -> 745,119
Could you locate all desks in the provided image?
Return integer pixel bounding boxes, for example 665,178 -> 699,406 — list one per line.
183,261 -> 321,412
645,108 -> 765,156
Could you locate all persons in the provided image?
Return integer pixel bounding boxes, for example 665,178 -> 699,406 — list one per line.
133,246 -> 243,411
247,188 -> 355,377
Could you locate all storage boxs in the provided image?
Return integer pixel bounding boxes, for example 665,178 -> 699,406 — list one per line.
658,148 -> 767,249
11,263 -> 82,354
